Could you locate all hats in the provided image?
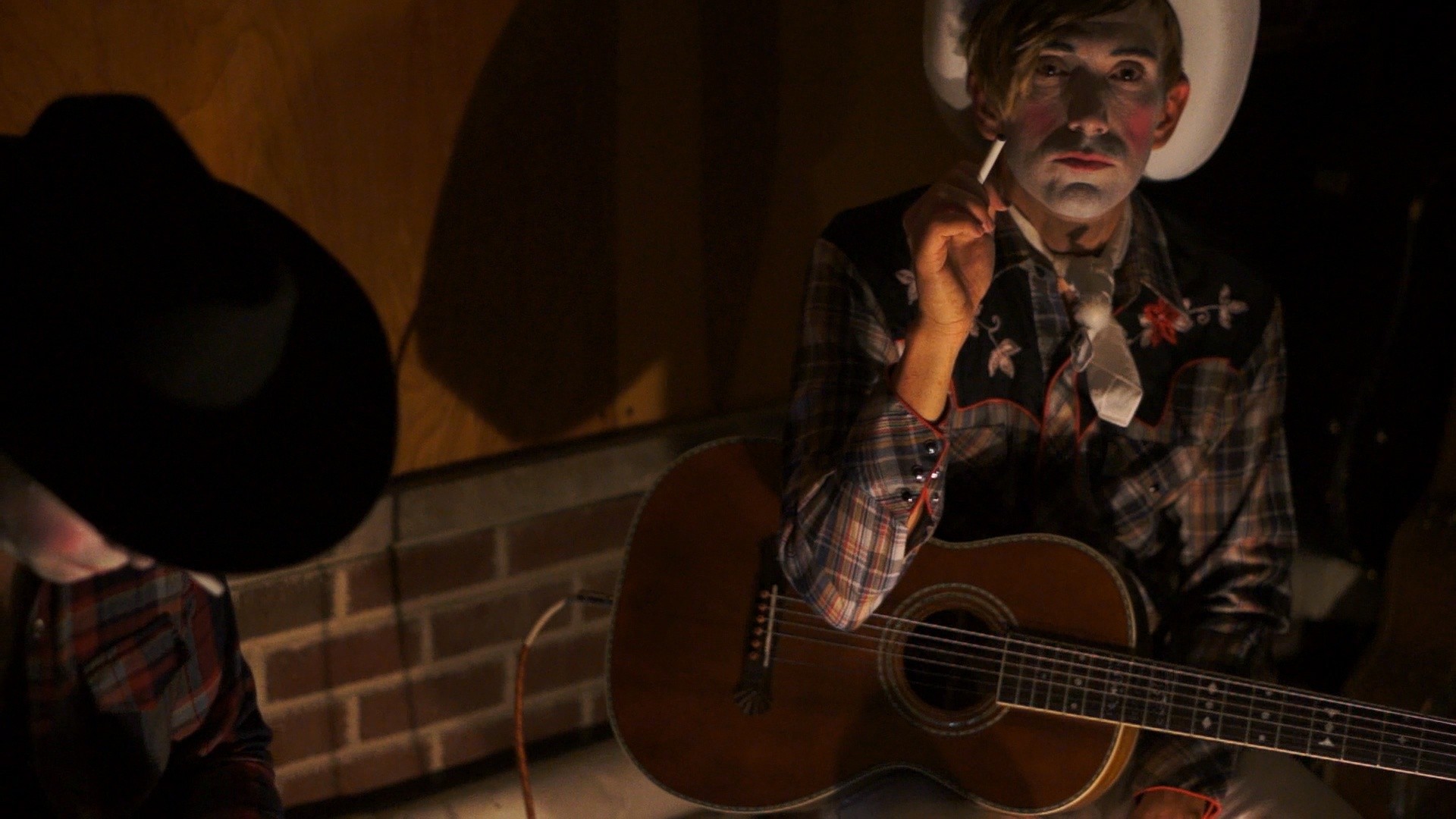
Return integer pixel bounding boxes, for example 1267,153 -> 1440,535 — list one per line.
0,93 -> 399,577
920,0 -> 1259,180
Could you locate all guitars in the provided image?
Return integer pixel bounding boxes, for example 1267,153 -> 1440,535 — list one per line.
604,433 -> 1456,817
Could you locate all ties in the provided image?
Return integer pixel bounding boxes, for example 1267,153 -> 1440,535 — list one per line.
994,183 -> 1143,428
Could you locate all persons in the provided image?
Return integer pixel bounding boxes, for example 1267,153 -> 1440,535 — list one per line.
0,91 -> 400,819
775,2 -> 1361,819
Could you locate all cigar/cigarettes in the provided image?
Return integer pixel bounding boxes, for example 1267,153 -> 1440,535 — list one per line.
977,133 -> 1006,185
